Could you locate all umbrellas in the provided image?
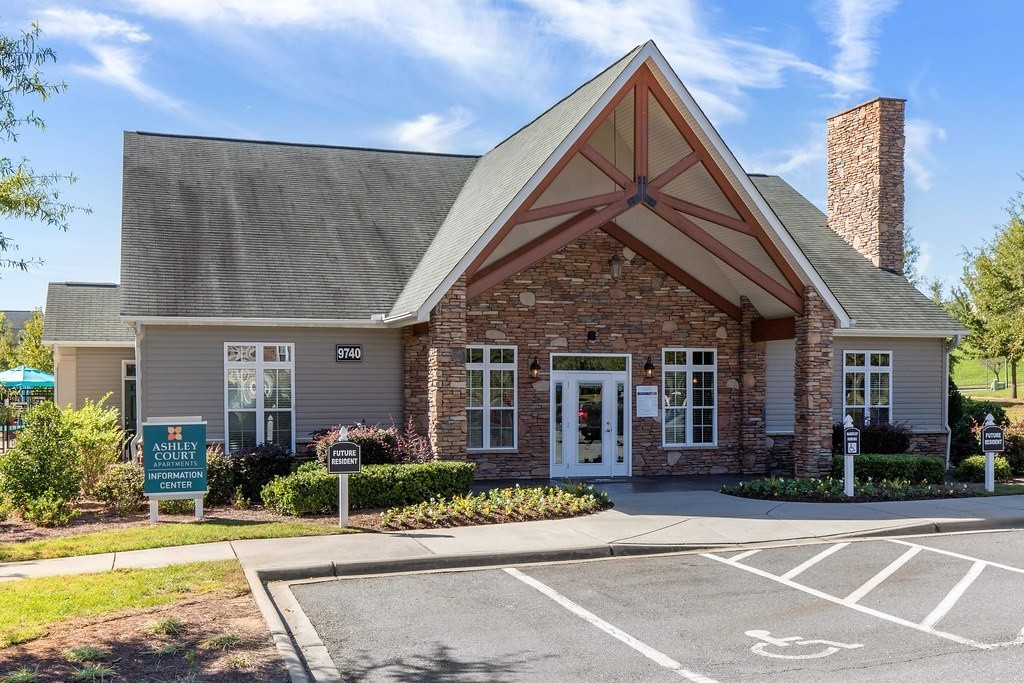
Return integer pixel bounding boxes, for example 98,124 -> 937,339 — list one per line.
0,365 -> 54,403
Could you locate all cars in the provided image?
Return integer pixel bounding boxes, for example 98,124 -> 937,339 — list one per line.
559,398 -> 623,437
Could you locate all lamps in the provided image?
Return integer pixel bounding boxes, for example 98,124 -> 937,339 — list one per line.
643,357 -> 655,379
587,330 -> 597,344
529,356 -> 543,378
609,108 -> 625,281
